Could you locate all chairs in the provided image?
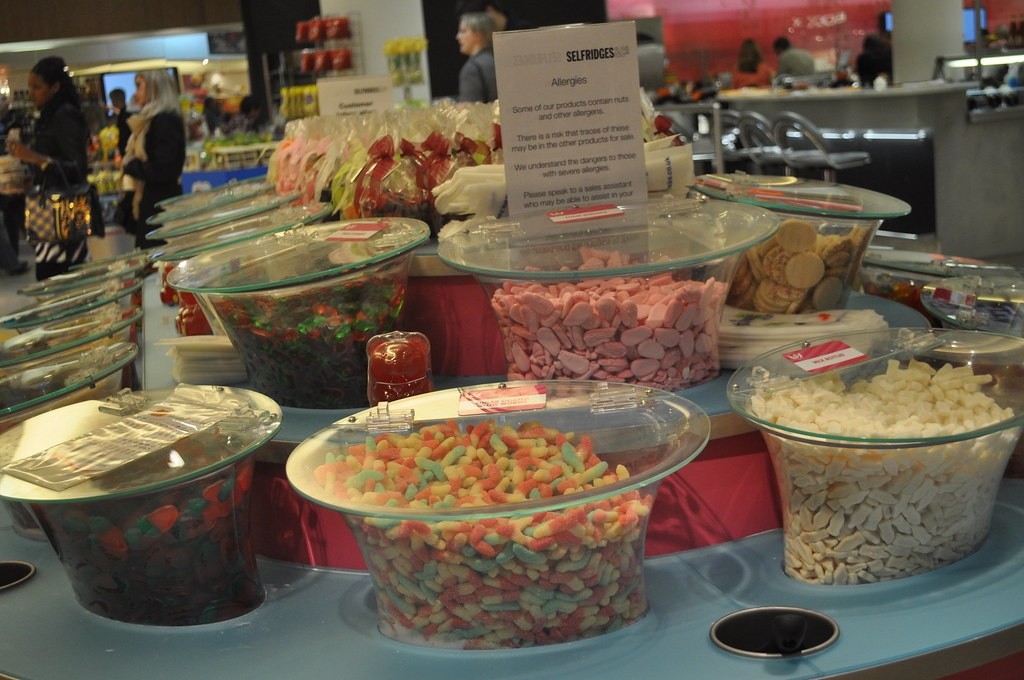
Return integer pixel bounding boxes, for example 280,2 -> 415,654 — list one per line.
772,111 -> 870,184
736,110 -> 797,177
712,110 -> 771,174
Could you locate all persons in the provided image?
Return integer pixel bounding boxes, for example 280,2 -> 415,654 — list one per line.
119,69 -> 187,282
454,1 -> 523,105
731,38 -> 772,90
770,37 -> 815,85
109,89 -> 131,159
0,93 -> 28,275
856,34 -> 892,86
5,56 -> 91,282
979,24 -> 1011,49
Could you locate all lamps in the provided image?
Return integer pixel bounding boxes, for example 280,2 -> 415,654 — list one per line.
931,56 -> 979,80
862,129 -> 932,142
819,128 -> 855,140
786,128 -> 805,141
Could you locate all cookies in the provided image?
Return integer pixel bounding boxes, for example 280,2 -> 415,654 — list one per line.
726,218 -> 875,317
492,244 -> 729,396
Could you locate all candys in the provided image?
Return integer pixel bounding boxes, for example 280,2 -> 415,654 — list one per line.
44,252 -> 657,648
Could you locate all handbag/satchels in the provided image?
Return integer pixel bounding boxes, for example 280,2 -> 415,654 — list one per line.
26,158 -> 91,243
111,172 -> 144,238
